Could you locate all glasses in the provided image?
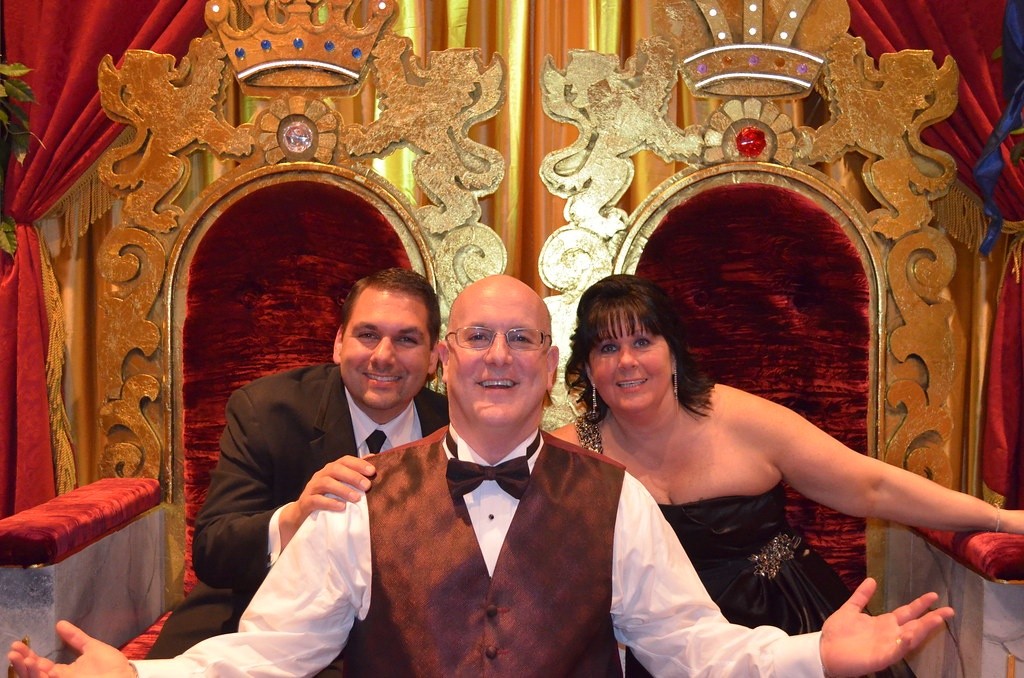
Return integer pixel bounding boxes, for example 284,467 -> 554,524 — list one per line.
445,324 -> 554,351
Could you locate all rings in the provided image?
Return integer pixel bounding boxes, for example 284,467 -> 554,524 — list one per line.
895,635 -> 901,646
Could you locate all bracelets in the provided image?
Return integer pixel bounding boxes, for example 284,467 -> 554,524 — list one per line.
994,502 -> 1000,532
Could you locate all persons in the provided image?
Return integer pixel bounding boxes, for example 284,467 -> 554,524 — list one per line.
143,267 -> 450,662
545,274 -> 1024,678
7,273 -> 954,678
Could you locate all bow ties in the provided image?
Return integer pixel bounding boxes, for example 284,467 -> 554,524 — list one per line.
446,427 -> 541,501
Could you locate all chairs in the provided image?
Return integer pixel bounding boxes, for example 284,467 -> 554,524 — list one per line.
609,155 -> 892,601
121,159 -> 441,662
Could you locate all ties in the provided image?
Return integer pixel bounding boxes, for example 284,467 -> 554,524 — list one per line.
362,430 -> 387,456
